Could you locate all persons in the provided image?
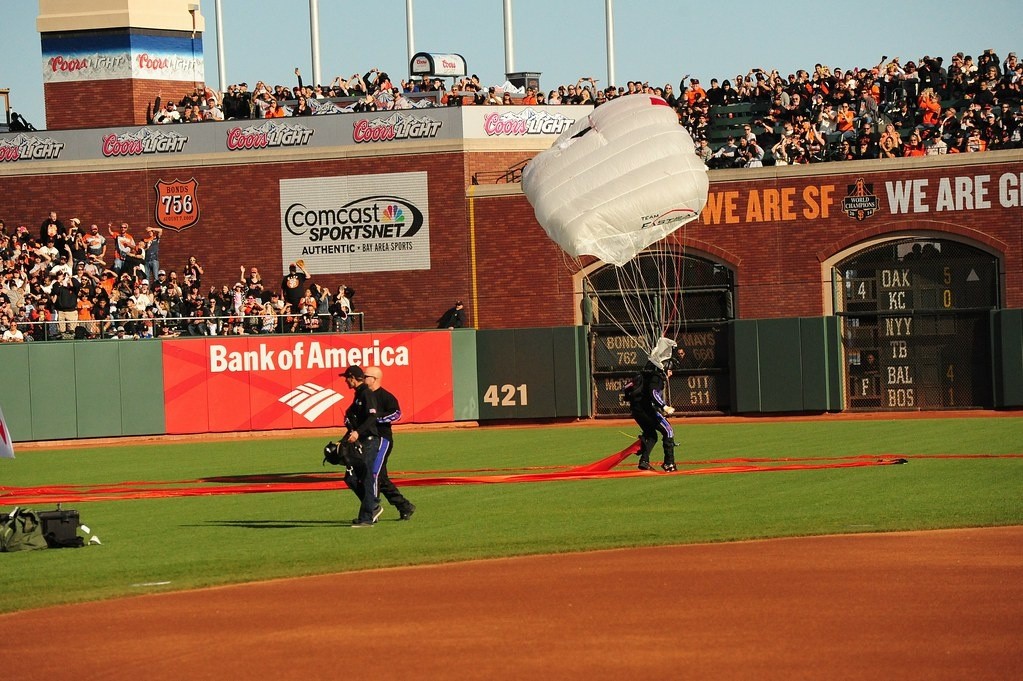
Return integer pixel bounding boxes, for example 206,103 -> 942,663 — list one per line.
151,49 -> 1023,170
436,301 -> 465,331
864,354 -> 878,371
673,349 -> 693,370
630,357 -> 677,473
336,365 -> 416,527
0,212 -> 355,342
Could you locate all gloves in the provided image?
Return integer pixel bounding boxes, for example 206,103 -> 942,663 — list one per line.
663,405 -> 675,415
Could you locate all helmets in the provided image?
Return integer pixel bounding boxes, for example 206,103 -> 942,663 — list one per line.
657,360 -> 674,376
324,441 -> 339,465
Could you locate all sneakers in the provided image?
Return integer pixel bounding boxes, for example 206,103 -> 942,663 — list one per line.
638,460 -> 655,470
351,519 -> 374,527
399,504 -> 416,521
373,505 -> 383,523
661,463 -> 678,472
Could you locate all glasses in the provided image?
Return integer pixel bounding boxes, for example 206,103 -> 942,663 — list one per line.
364,375 -> 373,379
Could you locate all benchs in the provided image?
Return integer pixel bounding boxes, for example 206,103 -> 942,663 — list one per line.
706,81 -> 1023,171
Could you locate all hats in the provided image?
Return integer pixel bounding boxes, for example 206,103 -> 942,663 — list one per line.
964,56 -> 971,60
338,365 -> 364,380
932,132 -> 940,138
158,270 -> 166,275
986,113 -> 994,119
691,79 -> 699,85
196,296 -> 201,301
142,279 -> 149,286
251,267 -> 258,273
70,218 -> 80,225
91,225 -> 98,230
455,300 -> 463,306
239,83 -> 248,88
